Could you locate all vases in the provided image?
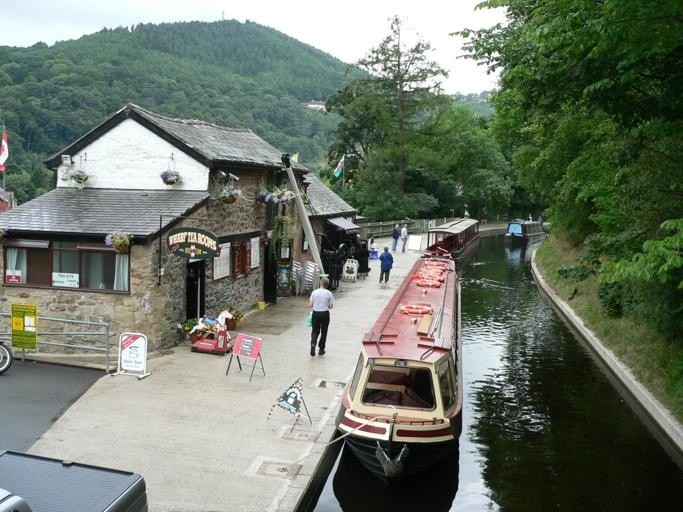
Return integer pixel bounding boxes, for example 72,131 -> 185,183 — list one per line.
162,176 -> 178,186
75,179 -> 86,184
221,195 -> 237,205
190,332 -> 202,344
226,319 -> 236,330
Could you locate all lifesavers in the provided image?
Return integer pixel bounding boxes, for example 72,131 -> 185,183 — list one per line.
417,261 -> 447,287
399,304 -> 432,313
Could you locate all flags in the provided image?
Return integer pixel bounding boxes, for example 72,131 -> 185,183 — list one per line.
332,153 -> 345,178
0,129 -> 10,173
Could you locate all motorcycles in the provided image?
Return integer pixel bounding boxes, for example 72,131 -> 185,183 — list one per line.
0,341 -> 12,374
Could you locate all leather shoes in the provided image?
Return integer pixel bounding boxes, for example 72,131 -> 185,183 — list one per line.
310,344 -> 314,356
319,350 -> 324,355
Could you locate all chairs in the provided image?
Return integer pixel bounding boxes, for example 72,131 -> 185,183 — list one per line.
341,258 -> 358,282
292,261 -> 321,296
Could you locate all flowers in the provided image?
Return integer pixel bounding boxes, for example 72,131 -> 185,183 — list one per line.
215,185 -> 243,198
223,304 -> 245,320
254,185 -> 296,206
160,170 -> 181,182
71,169 -> 90,179
105,228 -> 135,249
184,320 -> 207,332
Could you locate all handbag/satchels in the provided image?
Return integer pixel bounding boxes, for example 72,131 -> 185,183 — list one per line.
306,311 -> 313,327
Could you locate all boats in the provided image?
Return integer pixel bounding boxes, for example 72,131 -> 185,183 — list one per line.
504,218 -> 546,246
425,218 -> 481,269
336,250 -> 464,490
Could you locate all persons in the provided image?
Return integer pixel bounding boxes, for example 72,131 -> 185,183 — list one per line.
399,223 -> 407,254
537,215 -> 545,232
345,234 -> 374,281
378,246 -> 393,285
527,211 -> 532,221
307,276 -> 335,357
391,223 -> 401,253
